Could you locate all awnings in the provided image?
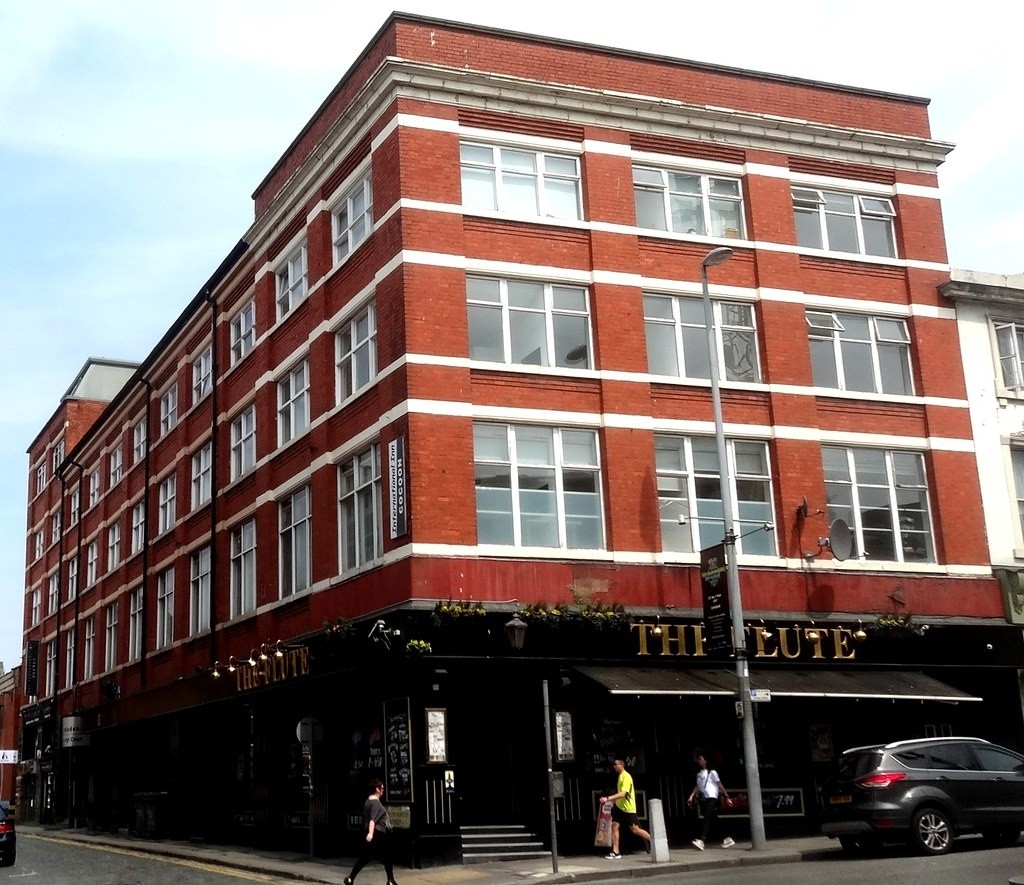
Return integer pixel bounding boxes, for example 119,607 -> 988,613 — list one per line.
573,664 -> 985,706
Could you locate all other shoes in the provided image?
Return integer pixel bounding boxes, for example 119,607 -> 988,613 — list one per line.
344,877 -> 353,885
387,880 -> 397,885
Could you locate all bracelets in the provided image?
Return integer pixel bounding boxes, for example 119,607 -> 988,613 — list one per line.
607,796 -> 609,801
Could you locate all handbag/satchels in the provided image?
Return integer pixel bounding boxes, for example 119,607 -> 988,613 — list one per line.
698,791 -> 706,803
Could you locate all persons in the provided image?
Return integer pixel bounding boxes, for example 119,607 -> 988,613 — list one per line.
811,727 -> 832,758
688,753 -> 737,850
600,758 -> 651,860
345,779 -> 399,885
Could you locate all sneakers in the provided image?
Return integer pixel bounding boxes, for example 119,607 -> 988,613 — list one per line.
604,851 -> 622,860
692,838 -> 704,851
721,839 -> 735,848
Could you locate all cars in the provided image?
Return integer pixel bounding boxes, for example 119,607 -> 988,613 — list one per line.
0,805 -> 16,867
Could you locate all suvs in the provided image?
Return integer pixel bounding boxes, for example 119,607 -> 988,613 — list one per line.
816,735 -> 1024,857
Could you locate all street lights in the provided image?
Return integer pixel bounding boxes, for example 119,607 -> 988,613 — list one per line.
700,247 -> 773,855
